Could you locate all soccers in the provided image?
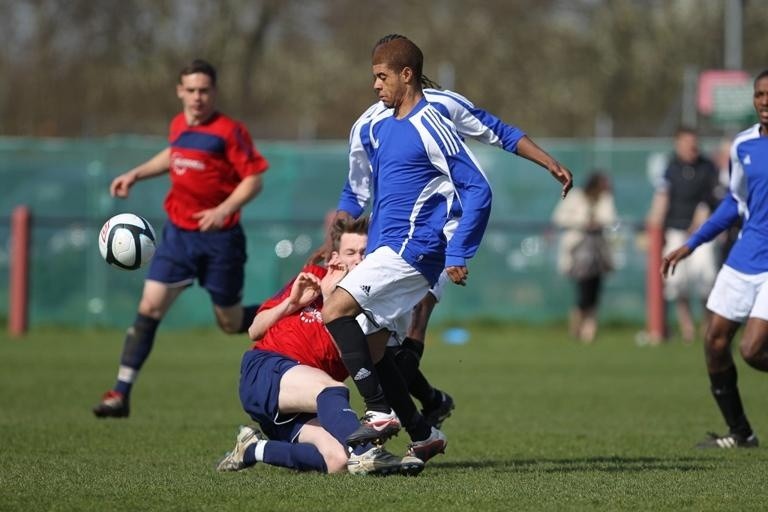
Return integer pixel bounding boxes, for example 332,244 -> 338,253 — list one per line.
98,213 -> 156,271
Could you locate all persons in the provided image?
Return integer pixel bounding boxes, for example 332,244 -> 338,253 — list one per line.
656,64 -> 767,452
300,33 -> 575,434
551,168 -> 623,347
213,212 -> 428,477
88,56 -> 264,421
318,38 -> 495,467
639,130 -> 742,346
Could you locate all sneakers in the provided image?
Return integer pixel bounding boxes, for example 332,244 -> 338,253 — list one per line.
344,388 -> 455,475
695,432 -> 758,446
216,425 -> 265,471
93,391 -> 128,416
